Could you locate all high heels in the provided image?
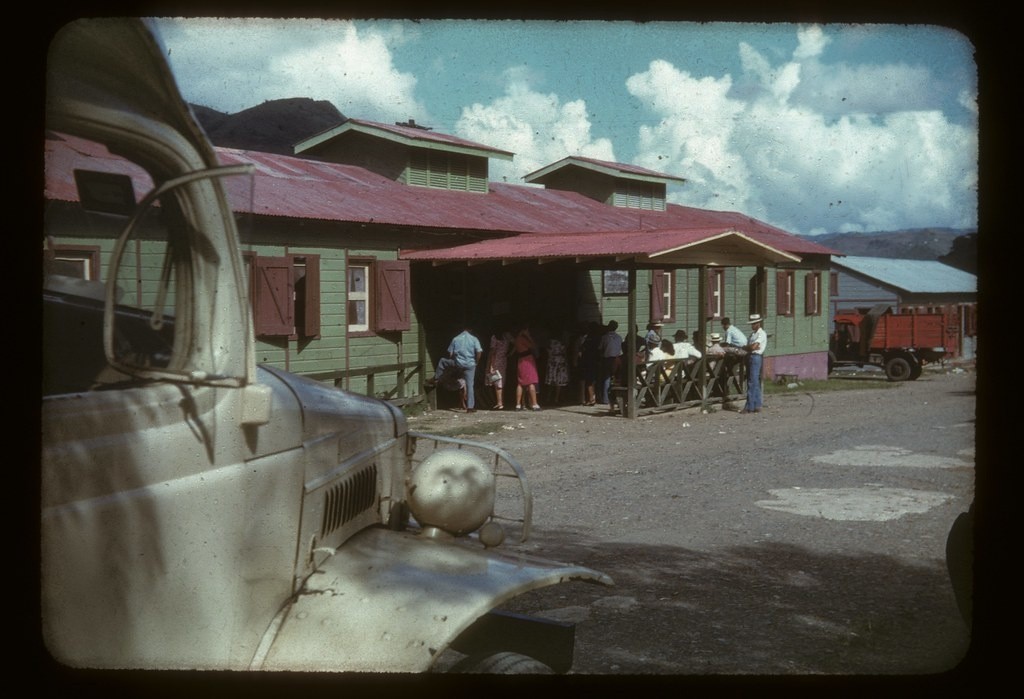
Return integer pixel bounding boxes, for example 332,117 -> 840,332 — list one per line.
584,400 -> 596,407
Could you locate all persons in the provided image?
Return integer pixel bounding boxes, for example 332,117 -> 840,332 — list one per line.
544,320 -> 724,416
429,326 -> 483,413
739,314 -> 767,414
515,322 -> 541,411
721,317 -> 747,347
484,323 -> 516,411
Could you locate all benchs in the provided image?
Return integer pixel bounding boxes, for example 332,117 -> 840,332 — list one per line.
608,354 -> 744,415
777,374 -> 798,384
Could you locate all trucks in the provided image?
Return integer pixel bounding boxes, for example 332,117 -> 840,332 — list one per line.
34,16 -> 616,681
826,302 -> 968,381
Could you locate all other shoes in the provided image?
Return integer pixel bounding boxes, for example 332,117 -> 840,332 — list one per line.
430,378 -> 439,384
515,405 -> 522,411
740,409 -> 760,414
532,405 -> 543,411
467,408 -> 477,412
491,405 -> 505,410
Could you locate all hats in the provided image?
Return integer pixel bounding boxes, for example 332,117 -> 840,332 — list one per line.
645,334 -> 662,345
672,330 -> 688,338
747,313 -> 762,324
711,333 -> 723,341
650,320 -> 665,327
